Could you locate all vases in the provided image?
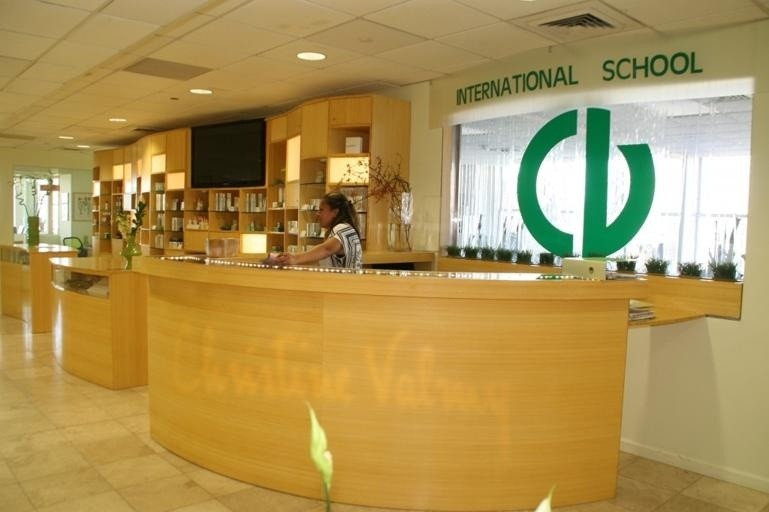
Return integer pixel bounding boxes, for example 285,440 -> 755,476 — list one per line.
120,238 -> 143,270
386,221 -> 413,252
28,217 -> 40,253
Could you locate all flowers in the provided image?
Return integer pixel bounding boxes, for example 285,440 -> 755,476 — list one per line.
11,170 -> 56,217
340,152 -> 425,251
112,200 -> 148,243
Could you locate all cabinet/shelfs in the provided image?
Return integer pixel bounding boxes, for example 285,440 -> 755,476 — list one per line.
90,94 -> 420,265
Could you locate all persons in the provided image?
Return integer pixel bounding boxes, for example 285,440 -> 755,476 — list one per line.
273,192 -> 363,269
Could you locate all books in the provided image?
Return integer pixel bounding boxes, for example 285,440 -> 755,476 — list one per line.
629,299 -> 655,321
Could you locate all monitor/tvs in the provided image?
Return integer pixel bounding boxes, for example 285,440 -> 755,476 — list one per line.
191,118 -> 266,188
